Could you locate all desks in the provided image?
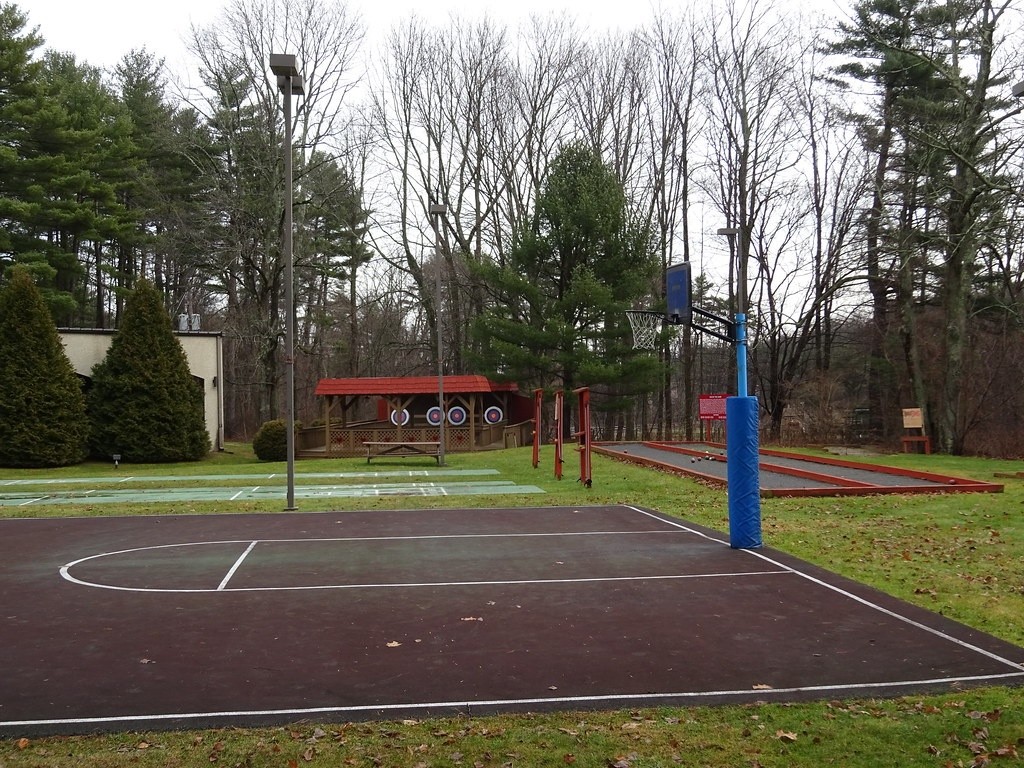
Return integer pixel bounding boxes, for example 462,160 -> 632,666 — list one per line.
362,442 -> 442,464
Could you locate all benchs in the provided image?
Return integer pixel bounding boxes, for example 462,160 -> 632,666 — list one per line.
363,451 -> 442,457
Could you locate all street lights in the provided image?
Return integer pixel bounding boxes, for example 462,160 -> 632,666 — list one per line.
717,227 -> 743,313
270,52 -> 305,510
430,205 -> 447,466
